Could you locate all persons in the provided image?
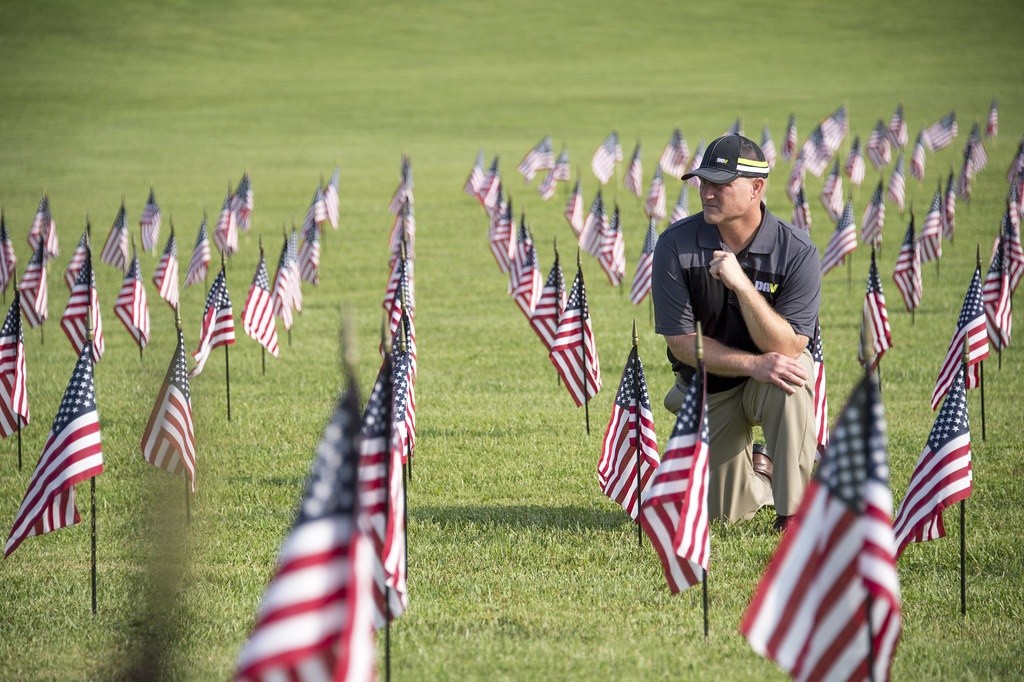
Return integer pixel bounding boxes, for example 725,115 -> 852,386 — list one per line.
652,134 -> 822,532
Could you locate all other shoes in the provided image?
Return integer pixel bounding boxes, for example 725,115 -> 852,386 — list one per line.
752,444 -> 773,482
770,513 -> 795,533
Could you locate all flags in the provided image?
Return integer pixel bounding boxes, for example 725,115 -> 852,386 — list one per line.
0,157 -> 414,682
460,98 -> 1023,682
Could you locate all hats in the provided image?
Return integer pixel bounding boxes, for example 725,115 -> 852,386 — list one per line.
681,132 -> 769,184
